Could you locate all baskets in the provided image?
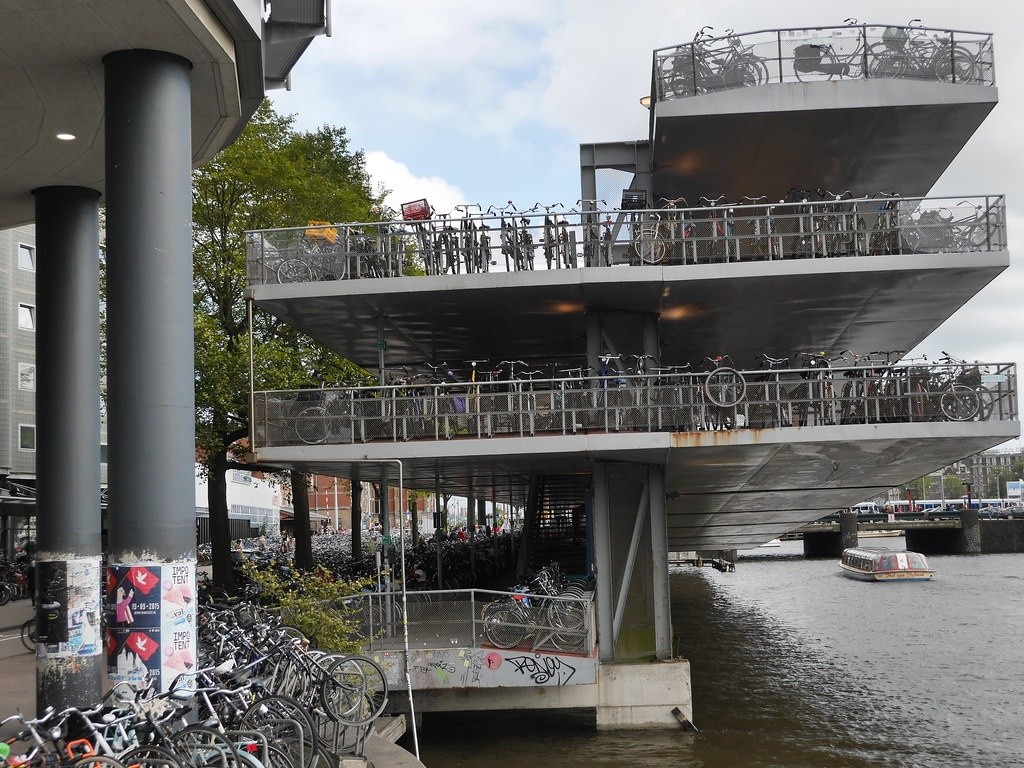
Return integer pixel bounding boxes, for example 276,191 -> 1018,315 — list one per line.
622,189 -> 647,209
670,367 -> 689,383
882,26 -> 907,51
305,221 -> 337,245
961,371 -> 982,386
401,199 -> 431,222
909,362 -> 928,380
297,385 -> 326,401
234,606 -> 256,629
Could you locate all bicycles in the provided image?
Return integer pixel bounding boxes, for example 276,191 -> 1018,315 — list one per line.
656,16 -> 985,99
263,345 -> 998,443
0,512 -> 597,767
263,188 -> 1012,284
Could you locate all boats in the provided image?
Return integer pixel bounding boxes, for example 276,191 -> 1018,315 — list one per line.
836,546 -> 937,581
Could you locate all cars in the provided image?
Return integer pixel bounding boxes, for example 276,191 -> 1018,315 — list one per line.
976,507 -> 1002,519
998,506 -> 1024,521
920,508 -> 934,516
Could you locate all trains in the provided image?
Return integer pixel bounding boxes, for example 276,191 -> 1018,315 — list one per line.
829,502 -> 880,528
883,499 -> 1024,510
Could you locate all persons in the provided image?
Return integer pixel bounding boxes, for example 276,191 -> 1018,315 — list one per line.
242,530 -> 288,549
418,526 -> 485,544
20,535 -> 37,554
915,505 -> 923,512
946,503 -> 955,520
